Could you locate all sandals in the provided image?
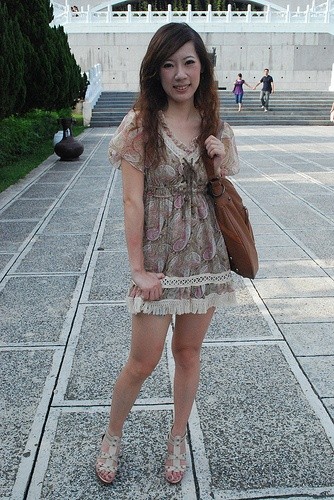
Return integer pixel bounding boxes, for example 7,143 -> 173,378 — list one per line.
96,431 -> 122,483
165,427 -> 186,483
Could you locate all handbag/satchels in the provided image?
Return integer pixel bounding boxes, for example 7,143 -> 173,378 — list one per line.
202,128 -> 258,279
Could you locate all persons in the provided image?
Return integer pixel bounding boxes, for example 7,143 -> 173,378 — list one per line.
253,68 -> 274,112
232,73 -> 256,112
96,22 -> 240,484
